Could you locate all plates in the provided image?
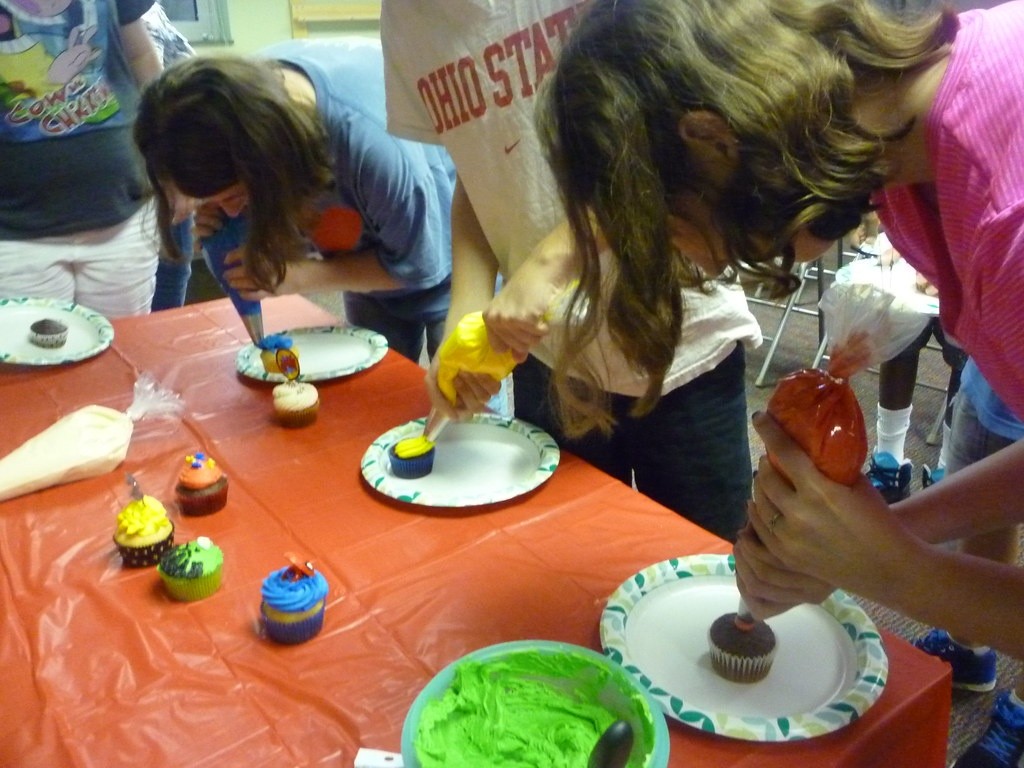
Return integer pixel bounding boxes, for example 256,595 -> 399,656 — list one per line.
599,554 -> 889,742
235,325 -> 388,383
0,295 -> 115,365
360,413 -> 561,507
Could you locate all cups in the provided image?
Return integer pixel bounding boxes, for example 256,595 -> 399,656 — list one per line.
400,640 -> 669,767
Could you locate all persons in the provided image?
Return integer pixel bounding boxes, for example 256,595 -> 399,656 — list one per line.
850,202 -> 970,503
535,0 -> 1024,768
0,0 -> 207,317
131,37 -> 510,419
141,0 -> 201,313
380,0 -> 764,544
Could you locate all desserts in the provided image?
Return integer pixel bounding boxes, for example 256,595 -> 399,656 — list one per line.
156,536 -> 224,601
386,435 -> 435,478
113,494 -> 175,568
174,453 -> 230,518
28,318 -> 69,349
260,552 -> 330,644
257,333 -> 300,373
707,611 -> 776,684
272,381 -> 320,427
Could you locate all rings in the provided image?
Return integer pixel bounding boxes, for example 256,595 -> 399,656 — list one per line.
765,512 -> 781,535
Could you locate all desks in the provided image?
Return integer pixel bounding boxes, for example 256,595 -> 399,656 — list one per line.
817,231 -> 958,446
0,293 -> 951,768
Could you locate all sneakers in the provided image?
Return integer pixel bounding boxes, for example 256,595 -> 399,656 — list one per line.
908,628 -> 996,692
949,688 -> 1024,768
863,445 -> 913,504
